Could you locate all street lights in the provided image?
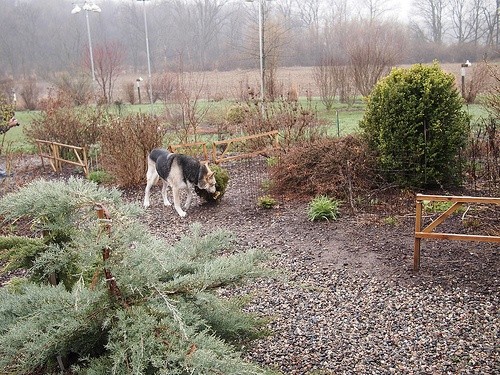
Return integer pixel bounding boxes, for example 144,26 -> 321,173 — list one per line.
71,0 -> 102,80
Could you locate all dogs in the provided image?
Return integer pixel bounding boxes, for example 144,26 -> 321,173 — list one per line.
143,148 -> 216,218
